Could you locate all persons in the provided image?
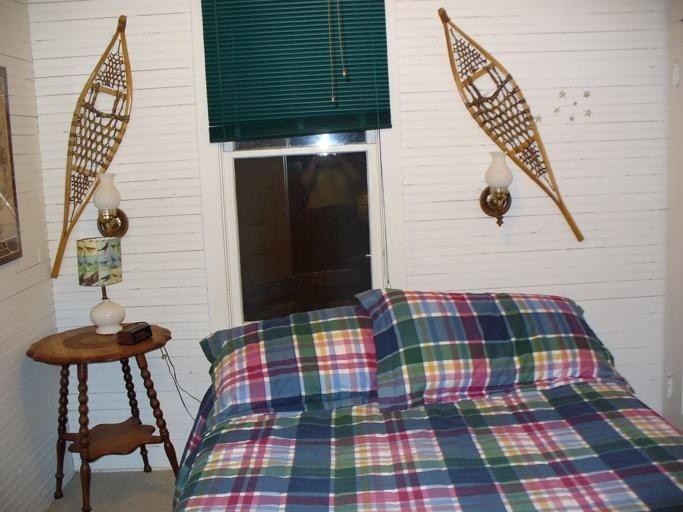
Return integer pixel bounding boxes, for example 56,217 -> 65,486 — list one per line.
298,152 -> 367,268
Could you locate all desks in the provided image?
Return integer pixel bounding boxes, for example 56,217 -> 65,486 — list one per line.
25,322 -> 179,512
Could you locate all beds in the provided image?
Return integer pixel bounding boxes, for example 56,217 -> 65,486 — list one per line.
176,288 -> 683,512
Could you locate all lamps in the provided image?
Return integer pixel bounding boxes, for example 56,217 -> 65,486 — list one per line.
92,173 -> 129,236
479,151 -> 514,227
76,238 -> 127,335
